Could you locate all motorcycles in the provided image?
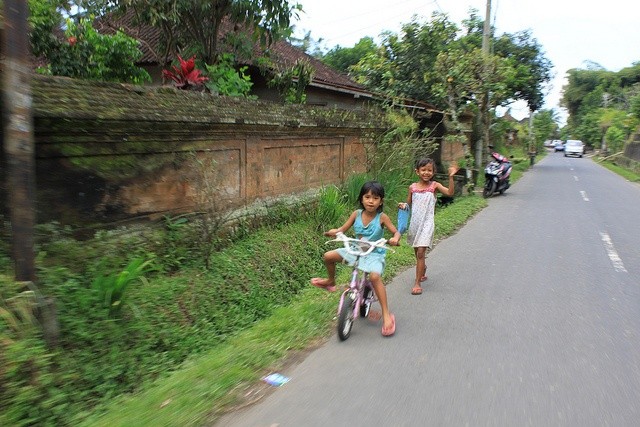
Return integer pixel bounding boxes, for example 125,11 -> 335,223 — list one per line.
483,145 -> 514,198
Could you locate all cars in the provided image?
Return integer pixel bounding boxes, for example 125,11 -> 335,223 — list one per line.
564,139 -> 586,158
555,142 -> 565,152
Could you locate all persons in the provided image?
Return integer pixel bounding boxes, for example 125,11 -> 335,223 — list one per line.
310,181 -> 402,337
397,157 -> 459,294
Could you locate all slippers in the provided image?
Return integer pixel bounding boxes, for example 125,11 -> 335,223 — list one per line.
411,287 -> 422,295
420,274 -> 427,282
382,314 -> 396,336
311,277 -> 338,292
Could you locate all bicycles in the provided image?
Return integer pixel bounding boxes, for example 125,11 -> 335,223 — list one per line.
325,231 -> 400,340
